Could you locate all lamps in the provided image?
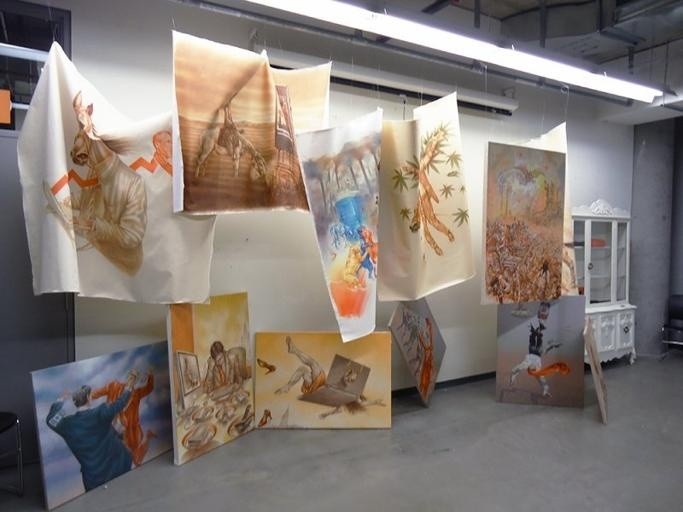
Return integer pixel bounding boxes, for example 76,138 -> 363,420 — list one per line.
249,45 -> 519,116
246,0 -> 677,103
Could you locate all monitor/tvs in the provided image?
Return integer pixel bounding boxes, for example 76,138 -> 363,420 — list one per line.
174,350 -> 204,410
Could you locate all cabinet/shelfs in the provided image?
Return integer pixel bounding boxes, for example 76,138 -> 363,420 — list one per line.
571,199 -> 637,365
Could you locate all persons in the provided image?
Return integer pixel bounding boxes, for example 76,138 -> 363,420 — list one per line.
46,366 -> 159,492
510,320 -> 550,398
203,340 -> 242,395
274,335 -> 387,420
152,129 -> 173,163
343,226 -> 378,289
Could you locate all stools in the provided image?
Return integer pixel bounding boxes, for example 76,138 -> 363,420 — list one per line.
0,412 -> 23,496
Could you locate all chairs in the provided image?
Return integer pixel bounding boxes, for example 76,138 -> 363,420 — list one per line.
661,295 -> 683,359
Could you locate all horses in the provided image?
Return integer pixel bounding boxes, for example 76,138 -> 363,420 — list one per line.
193,126 -> 268,178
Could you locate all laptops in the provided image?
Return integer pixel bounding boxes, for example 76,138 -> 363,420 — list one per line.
298,352 -> 371,409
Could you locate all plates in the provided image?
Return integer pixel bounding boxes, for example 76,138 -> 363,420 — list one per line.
181,406 -> 253,451
